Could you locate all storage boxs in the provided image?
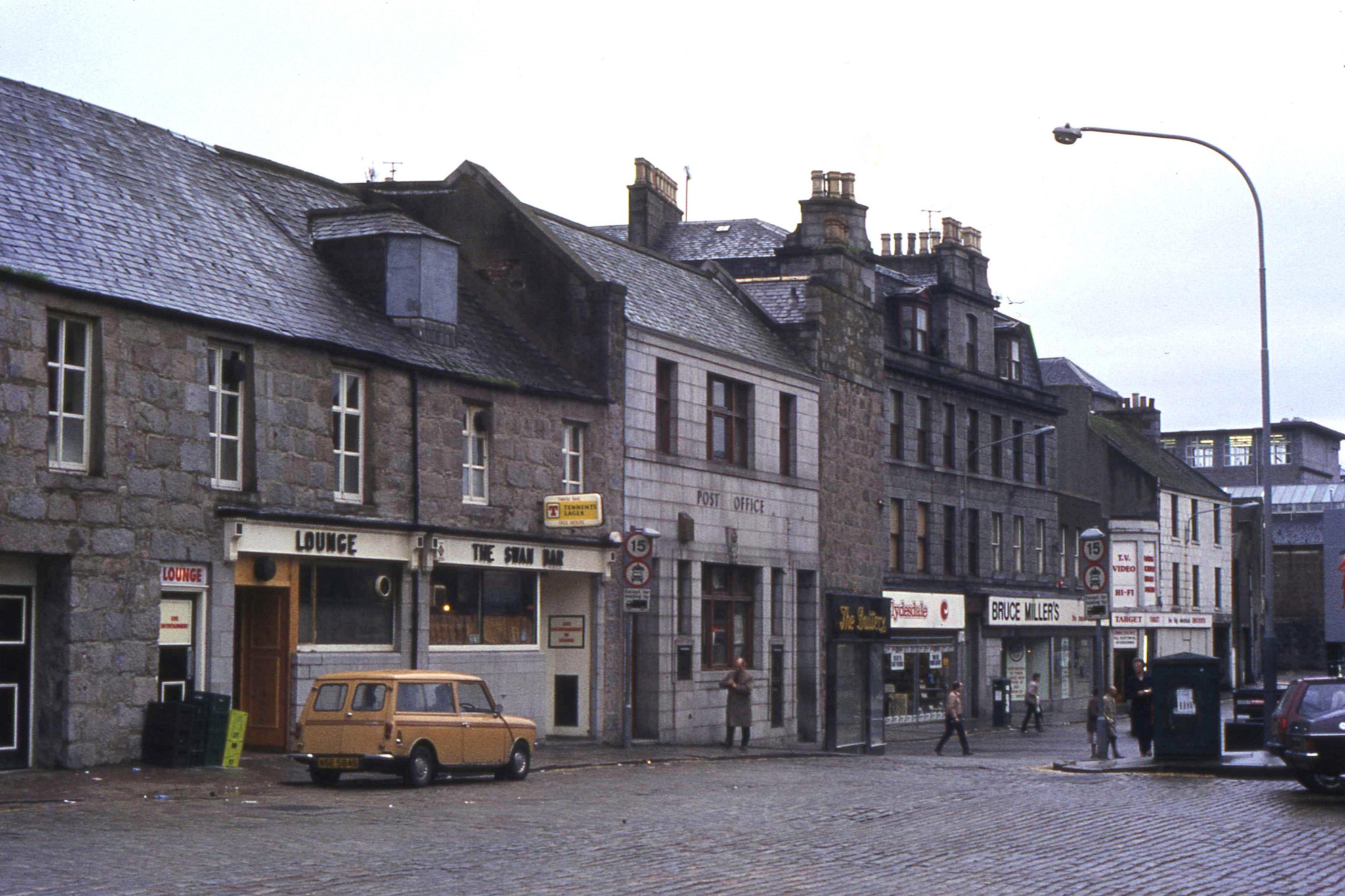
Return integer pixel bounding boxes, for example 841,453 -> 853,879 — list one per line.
142,691 -> 248,767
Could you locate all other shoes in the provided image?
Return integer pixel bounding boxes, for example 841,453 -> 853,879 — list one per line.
720,743 -> 732,748
740,746 -> 747,751
935,749 -> 945,756
963,751 -> 974,755
1020,730 -> 1028,734
1037,728 -> 1047,733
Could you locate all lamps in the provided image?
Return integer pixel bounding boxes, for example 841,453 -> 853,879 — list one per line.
1185,502 -> 1260,544
959,425 -> 1056,513
629,525 -> 662,538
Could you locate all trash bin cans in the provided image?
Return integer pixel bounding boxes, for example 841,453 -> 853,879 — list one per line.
992,679 -> 1012,726
143,691 -> 249,767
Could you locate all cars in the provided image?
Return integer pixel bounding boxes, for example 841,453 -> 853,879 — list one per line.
293,667 -> 537,788
1266,674 -> 1345,795
1233,673 -> 1324,720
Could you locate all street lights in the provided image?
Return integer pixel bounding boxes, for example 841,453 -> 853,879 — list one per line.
1078,528 -> 1105,697
1053,121 -> 1282,744
624,523 -> 663,758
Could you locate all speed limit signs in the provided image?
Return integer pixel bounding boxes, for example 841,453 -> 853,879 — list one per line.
1082,542 -> 1106,563
624,531 -> 653,562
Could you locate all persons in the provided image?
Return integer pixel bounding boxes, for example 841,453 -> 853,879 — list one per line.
1103,685 -> 1124,758
718,658 -> 753,751
1086,688 -> 1104,758
933,682 -> 973,756
1124,658 -> 1155,756
1021,673 -> 1046,734
